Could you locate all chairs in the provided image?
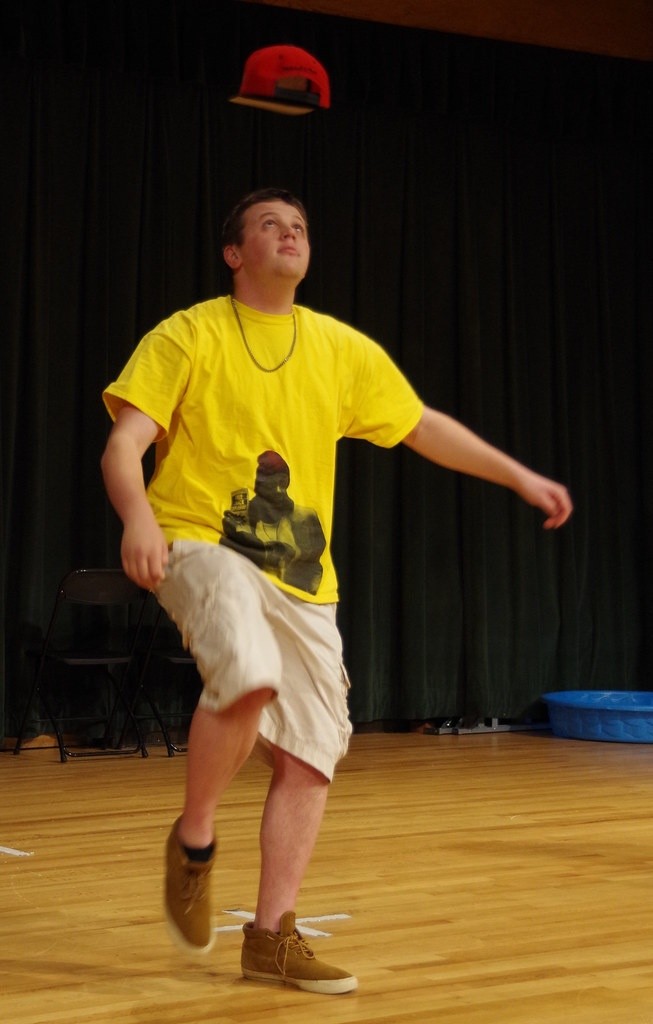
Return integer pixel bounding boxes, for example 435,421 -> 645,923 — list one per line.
117,606 -> 197,757
13,569 -> 148,763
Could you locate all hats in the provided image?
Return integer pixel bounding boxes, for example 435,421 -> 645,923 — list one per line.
229,45 -> 331,114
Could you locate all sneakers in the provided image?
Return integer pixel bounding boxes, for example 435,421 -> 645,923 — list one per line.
241,911 -> 358,994
164,813 -> 217,954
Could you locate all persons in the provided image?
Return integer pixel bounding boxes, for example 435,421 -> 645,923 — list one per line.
102,185 -> 573,998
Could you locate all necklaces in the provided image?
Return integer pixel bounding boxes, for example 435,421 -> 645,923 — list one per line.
230,294 -> 296,372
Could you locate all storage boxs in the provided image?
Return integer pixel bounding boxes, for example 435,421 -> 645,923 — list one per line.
539,689 -> 653,743
